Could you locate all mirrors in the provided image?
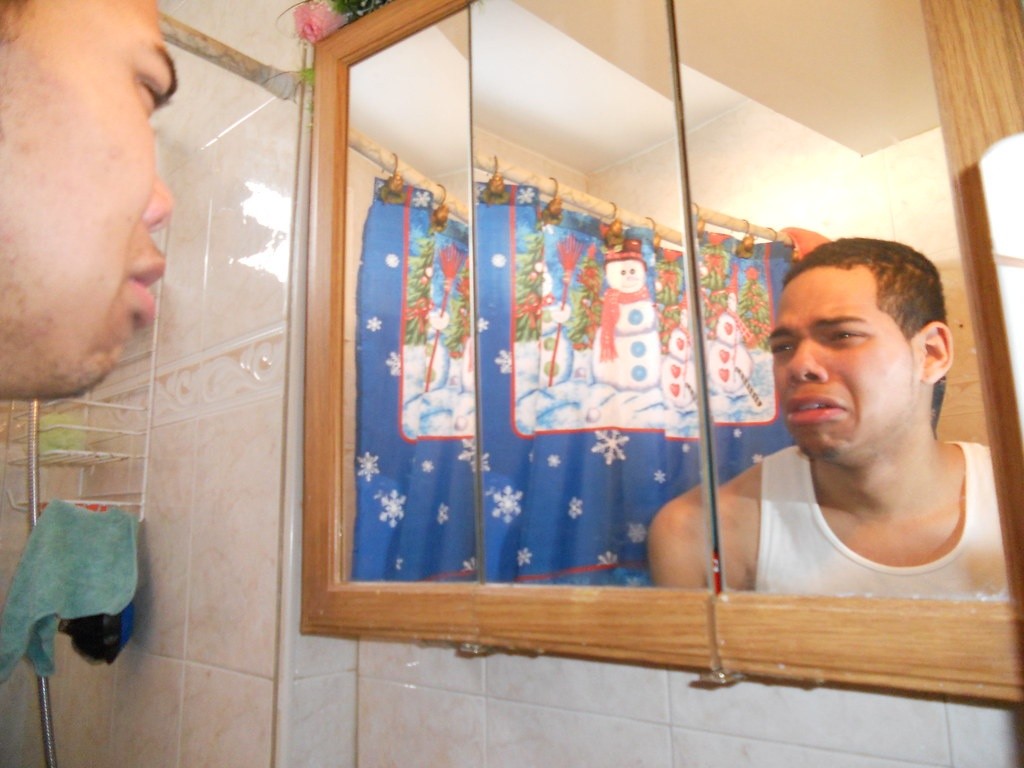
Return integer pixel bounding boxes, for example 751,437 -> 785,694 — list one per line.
305,0 -> 1024,704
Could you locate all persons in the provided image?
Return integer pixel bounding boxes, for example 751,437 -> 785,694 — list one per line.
645,237 -> 1012,600
0,3 -> 178,409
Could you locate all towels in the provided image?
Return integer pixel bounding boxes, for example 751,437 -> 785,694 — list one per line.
0,497 -> 139,680
